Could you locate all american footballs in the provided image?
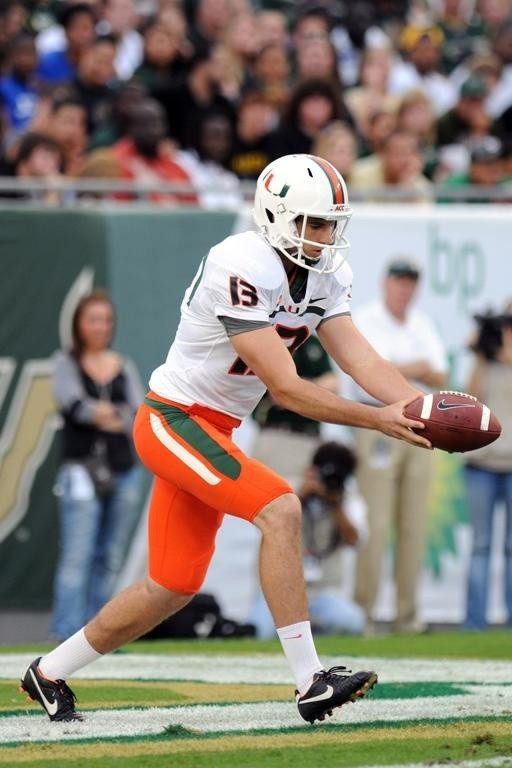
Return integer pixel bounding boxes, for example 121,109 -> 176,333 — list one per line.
402,390 -> 502,452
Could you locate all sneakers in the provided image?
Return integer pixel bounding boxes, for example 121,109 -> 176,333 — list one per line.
19,656 -> 87,724
206,617 -> 255,638
293,665 -> 379,725
391,620 -> 430,634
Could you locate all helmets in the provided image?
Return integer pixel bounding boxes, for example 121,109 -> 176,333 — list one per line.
251,151 -> 354,275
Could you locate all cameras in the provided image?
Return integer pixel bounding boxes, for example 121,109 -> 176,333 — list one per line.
319,462 -> 346,494
